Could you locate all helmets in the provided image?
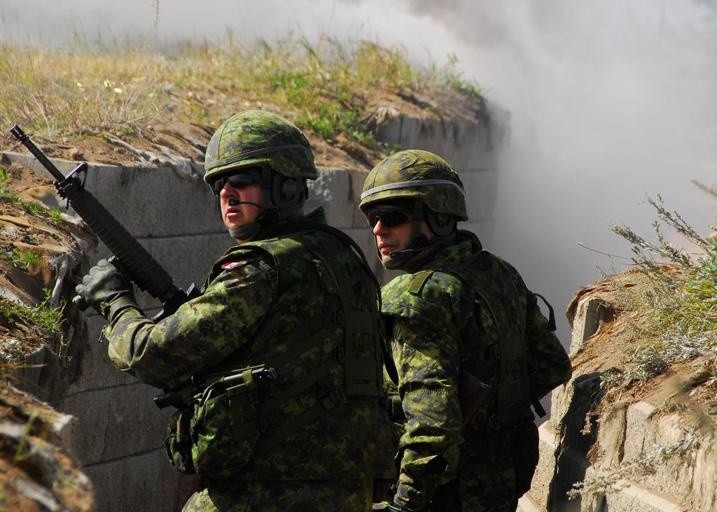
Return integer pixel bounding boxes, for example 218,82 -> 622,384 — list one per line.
358,150 -> 468,222
203,110 -> 319,184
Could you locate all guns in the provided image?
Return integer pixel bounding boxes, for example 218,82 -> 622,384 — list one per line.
9,124 -> 237,414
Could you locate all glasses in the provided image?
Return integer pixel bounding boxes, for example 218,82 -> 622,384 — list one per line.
364,207 -> 412,228
212,172 -> 270,195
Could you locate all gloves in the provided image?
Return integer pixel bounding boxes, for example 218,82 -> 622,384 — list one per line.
75,258 -> 134,323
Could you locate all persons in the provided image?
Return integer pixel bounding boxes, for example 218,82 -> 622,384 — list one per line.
71,109 -> 400,511
357,147 -> 575,510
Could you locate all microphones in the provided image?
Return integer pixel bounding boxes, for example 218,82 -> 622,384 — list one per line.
389,248 -> 424,258
228,198 -> 263,210
